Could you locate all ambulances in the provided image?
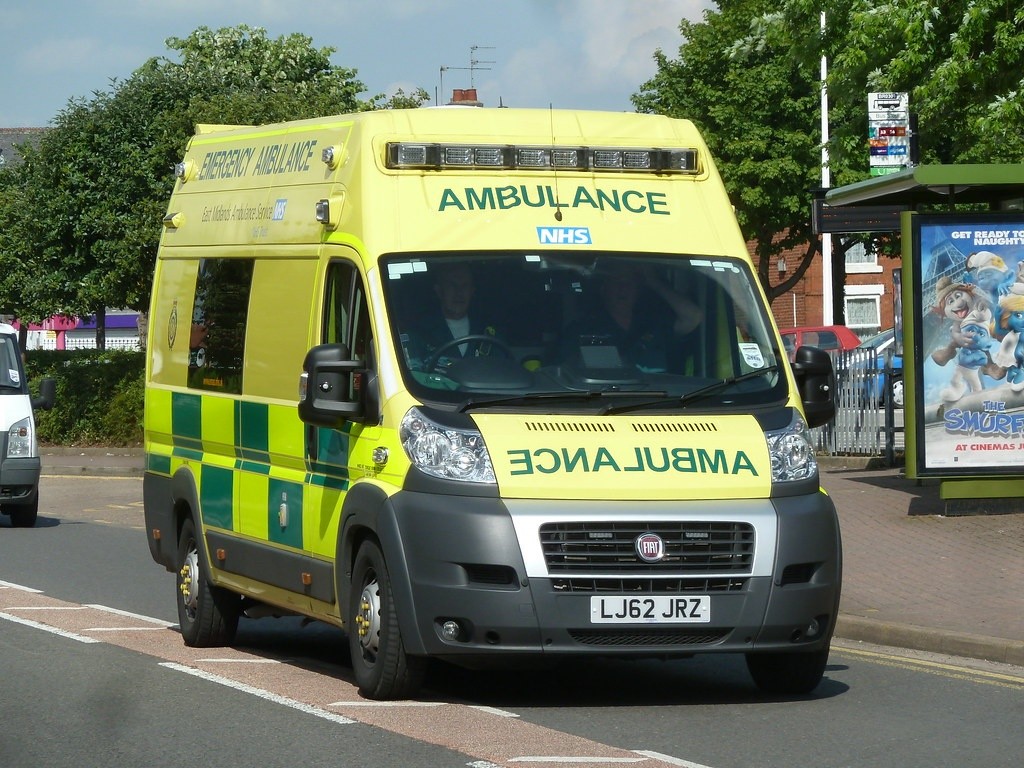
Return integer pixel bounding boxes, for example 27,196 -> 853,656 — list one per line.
137,104 -> 844,701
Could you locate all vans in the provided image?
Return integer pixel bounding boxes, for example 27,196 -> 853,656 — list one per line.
1,326 -> 40,525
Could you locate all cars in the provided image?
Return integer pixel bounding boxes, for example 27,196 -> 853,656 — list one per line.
776,320 -> 903,410
